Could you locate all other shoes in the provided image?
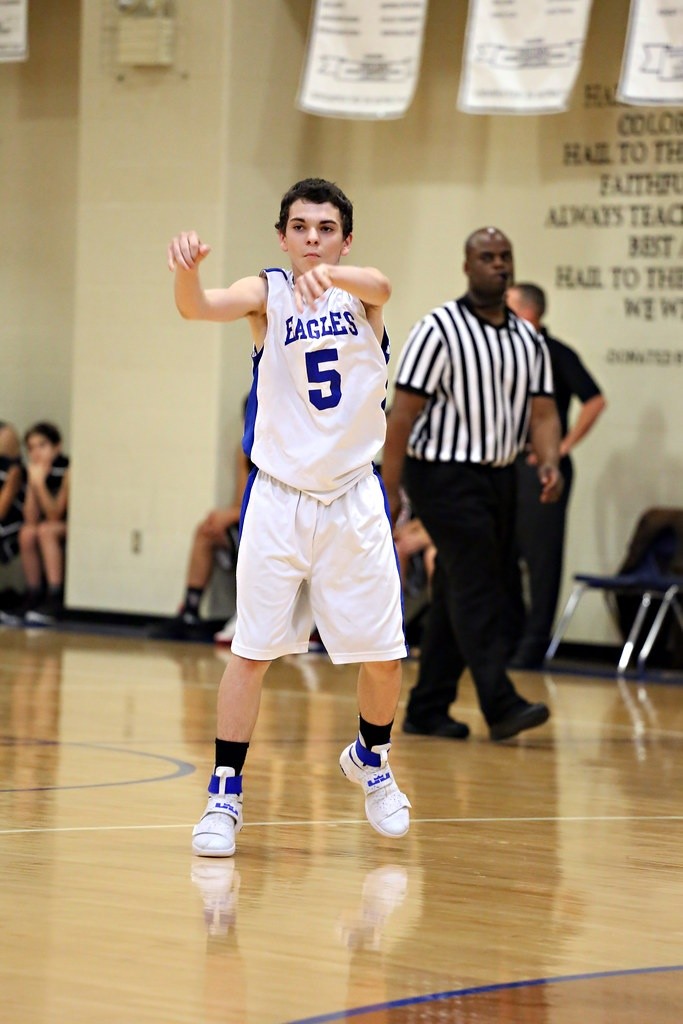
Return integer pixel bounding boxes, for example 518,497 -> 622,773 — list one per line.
27,608 -> 63,626
150,618 -> 202,640
215,609 -> 237,641
490,702 -> 549,740
403,714 -> 470,738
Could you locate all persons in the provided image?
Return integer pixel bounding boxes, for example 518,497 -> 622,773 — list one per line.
0,421 -> 71,627
149,395 -> 437,664
506,283 -> 607,675
166,176 -> 414,857
381,226 -> 565,741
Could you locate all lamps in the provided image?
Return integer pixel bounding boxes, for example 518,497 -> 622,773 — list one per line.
115,0 -> 174,66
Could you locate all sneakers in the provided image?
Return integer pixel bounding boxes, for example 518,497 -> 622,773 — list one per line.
339,726 -> 412,840
192,766 -> 245,857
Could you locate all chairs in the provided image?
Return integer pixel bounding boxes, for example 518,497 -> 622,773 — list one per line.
546,522 -> 683,679
